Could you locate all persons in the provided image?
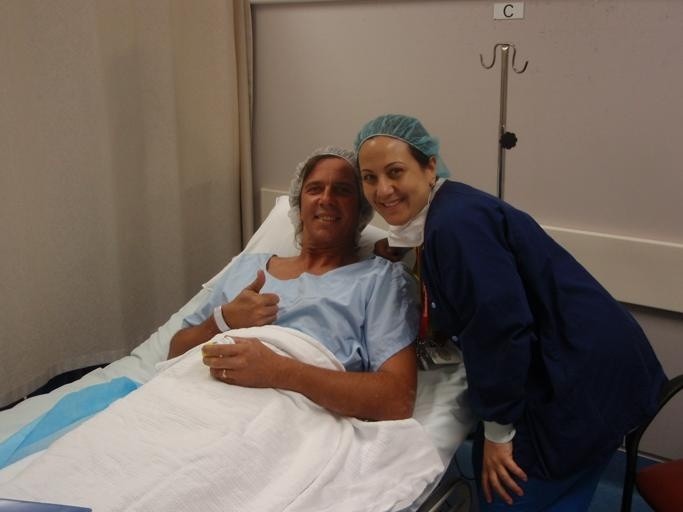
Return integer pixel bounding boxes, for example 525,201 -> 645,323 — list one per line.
166,143 -> 419,423
353,113 -> 670,512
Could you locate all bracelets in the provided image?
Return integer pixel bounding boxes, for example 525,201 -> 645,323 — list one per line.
214,306 -> 232,334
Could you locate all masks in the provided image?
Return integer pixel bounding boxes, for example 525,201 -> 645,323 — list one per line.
388,206 -> 429,249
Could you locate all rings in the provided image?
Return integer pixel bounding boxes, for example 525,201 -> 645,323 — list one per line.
222,371 -> 227,381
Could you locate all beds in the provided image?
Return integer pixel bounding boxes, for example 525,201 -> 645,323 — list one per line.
0,195 -> 481,512
